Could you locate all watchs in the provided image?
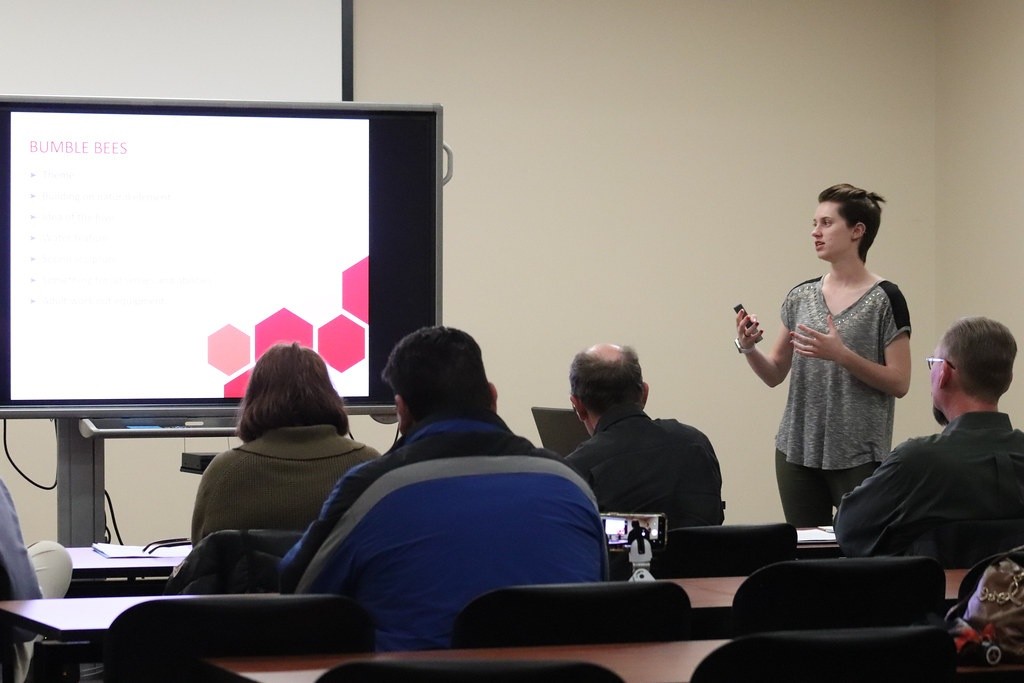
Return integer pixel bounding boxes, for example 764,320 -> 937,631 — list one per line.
734,337 -> 755,354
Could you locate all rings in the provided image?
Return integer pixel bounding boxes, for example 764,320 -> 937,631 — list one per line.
742,330 -> 751,337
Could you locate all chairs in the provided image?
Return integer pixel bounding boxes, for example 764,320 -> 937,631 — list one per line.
0,528 -> 1024,683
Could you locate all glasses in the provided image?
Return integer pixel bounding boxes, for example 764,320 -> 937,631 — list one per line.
926,356 -> 956,371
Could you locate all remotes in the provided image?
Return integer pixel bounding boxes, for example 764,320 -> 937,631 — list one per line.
734,304 -> 763,344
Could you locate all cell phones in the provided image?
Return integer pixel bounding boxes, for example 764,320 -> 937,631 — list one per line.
596,512 -> 668,552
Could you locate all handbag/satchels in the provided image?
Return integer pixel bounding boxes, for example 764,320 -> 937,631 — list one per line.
962,553 -> 1024,656
946,617 -> 1001,665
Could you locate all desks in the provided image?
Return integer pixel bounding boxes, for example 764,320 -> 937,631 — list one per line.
62,546 -> 189,594
200,637 -> 842,683
1,562 -> 977,641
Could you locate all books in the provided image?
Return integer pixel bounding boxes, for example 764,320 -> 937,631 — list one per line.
797,525 -> 838,544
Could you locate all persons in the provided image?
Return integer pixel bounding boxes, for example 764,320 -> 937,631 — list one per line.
561,343 -> 726,529
279,325 -> 611,654
186,342 -> 382,558
830,316 -> 1024,559
735,184 -> 912,529
626,519 -> 650,545
